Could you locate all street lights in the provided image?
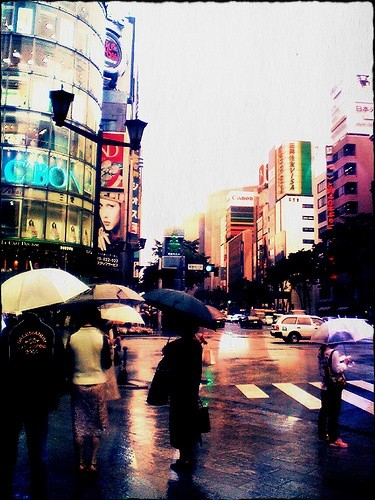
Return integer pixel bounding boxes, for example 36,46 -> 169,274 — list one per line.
48,83 -> 149,283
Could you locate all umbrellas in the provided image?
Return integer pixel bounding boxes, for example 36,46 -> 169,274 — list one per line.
143,289 -> 227,331
310,315 -> 374,357
0,267 -> 147,326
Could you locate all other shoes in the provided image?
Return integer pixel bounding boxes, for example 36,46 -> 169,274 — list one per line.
176,458 -> 197,466
317,434 -> 329,440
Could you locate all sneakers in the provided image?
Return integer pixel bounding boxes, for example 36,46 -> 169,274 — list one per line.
328,438 -> 349,448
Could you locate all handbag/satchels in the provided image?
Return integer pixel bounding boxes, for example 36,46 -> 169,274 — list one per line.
324,349 -> 348,387
146,356 -> 172,406
100,335 -> 121,401
197,396 -> 211,434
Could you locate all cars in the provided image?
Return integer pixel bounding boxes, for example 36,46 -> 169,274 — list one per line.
321,317 -> 337,322
263,315 -> 274,325
226,313 -> 263,329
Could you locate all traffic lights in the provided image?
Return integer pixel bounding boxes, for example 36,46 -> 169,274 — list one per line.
204,263 -> 216,273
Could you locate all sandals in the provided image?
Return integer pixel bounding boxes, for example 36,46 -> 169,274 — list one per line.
90,463 -> 97,472
79,462 -> 89,471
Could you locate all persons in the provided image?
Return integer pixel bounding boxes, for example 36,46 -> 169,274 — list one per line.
84,229 -> 89,246
50,222 -> 60,240
98,198 -> 123,252
318,335 -> 354,449
0,308 -> 67,500
53,306 -> 123,471
161,317 -> 203,473
70,226 -> 76,243
101,160 -> 123,187
28,220 -> 37,237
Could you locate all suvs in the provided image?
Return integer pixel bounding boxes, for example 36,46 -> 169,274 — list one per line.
269,314 -> 326,344
